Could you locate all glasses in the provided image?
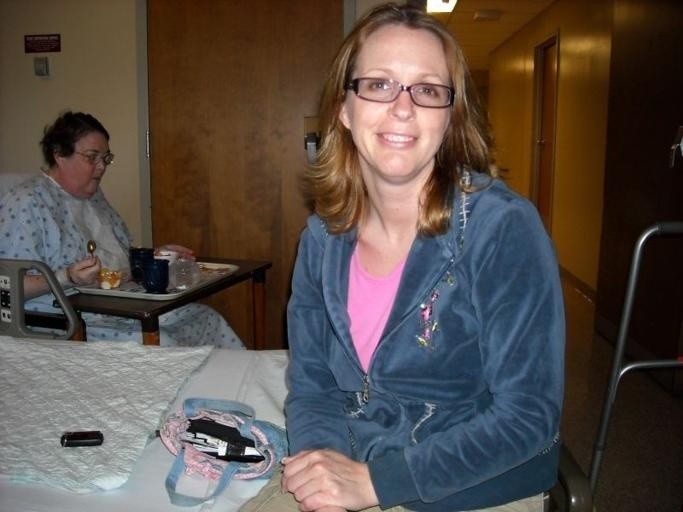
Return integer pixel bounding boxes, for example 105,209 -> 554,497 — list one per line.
344,77 -> 454,108
75,152 -> 113,164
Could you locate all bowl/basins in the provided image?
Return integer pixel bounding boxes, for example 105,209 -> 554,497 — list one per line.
96,270 -> 120,289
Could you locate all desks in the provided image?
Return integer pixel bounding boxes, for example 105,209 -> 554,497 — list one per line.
52,257 -> 272,350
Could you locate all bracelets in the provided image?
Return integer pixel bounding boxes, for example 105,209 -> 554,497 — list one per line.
54,269 -> 75,289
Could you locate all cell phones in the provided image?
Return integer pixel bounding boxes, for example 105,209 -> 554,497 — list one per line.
61,431 -> 103,447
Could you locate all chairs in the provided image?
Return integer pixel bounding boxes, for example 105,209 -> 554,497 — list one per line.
0,171 -> 86,341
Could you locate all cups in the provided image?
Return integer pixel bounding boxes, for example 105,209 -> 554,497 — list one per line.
129,247 -> 201,294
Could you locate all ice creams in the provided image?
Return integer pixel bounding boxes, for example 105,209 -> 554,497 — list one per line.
96,268 -> 122,289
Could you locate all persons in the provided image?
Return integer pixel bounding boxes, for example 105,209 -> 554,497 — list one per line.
0,112 -> 247,350
237,3 -> 567,512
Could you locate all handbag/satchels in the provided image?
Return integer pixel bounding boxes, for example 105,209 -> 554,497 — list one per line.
160,399 -> 289,506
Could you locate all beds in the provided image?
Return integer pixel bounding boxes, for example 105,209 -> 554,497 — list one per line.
0,335 -> 289,512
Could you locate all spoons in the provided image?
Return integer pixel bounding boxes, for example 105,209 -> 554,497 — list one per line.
86,240 -> 96,258
